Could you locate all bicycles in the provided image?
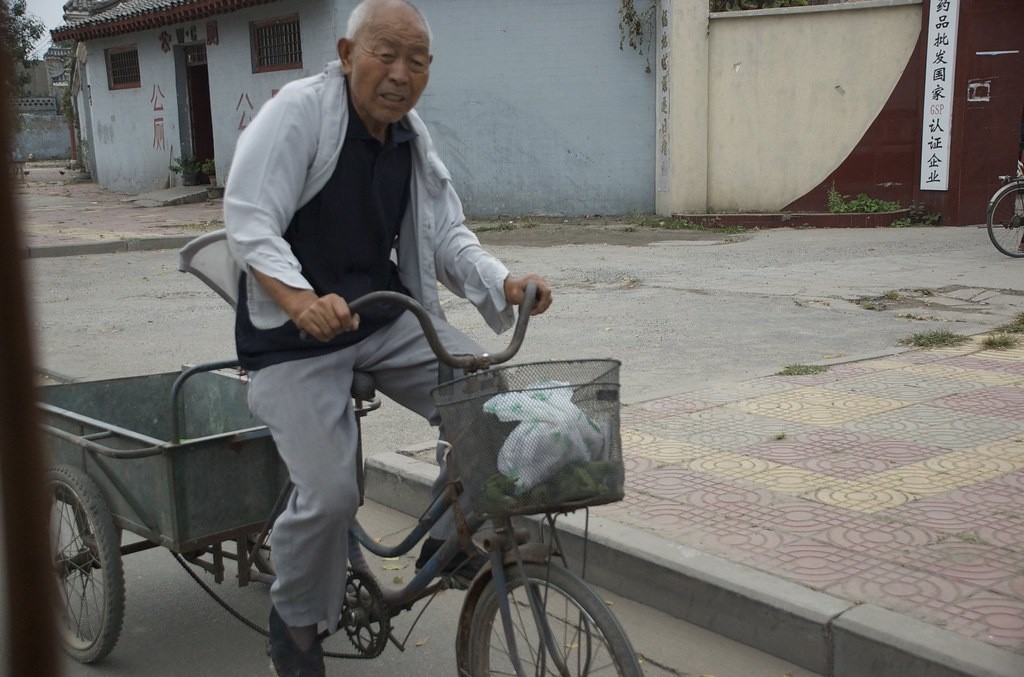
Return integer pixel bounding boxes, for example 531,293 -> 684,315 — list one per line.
986,174 -> 1024,258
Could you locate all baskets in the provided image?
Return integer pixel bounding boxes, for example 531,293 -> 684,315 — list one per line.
430,357 -> 624,519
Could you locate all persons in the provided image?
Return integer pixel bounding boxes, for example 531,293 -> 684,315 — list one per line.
178,0 -> 552,677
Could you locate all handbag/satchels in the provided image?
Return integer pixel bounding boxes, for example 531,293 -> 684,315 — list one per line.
483,381 -> 608,493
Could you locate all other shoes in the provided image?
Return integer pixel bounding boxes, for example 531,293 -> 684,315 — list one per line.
266,607 -> 326,677
415,539 -> 491,586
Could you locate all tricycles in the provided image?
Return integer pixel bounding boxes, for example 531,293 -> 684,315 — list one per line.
33,280 -> 648,677
